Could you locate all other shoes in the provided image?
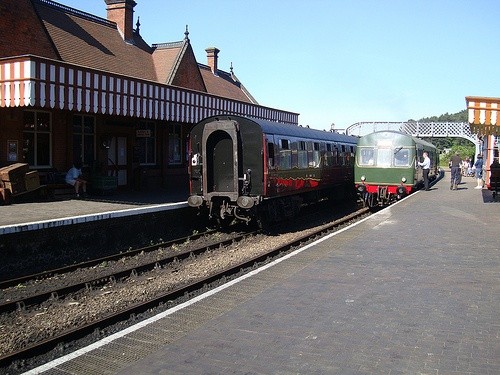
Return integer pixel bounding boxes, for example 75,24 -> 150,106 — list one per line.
450,187 -> 453,190
474,186 -> 482,189
425,189 -> 430,191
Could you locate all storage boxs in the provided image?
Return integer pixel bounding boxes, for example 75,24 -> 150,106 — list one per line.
91,176 -> 118,190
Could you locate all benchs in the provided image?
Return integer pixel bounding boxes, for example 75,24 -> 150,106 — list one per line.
40,184 -> 82,195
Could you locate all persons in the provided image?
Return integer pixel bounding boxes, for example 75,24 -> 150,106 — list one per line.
464,157 -> 473,177
474,154 -> 484,189
65,160 -> 89,197
418,152 -> 431,191
449,153 -> 462,190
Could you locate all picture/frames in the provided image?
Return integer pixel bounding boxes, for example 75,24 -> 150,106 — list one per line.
7,140 -> 18,162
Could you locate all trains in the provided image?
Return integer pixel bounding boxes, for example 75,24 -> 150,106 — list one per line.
186,114 -> 362,234
352,129 -> 443,214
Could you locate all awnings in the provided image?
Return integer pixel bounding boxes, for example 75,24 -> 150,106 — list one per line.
466,97 -> 500,136
